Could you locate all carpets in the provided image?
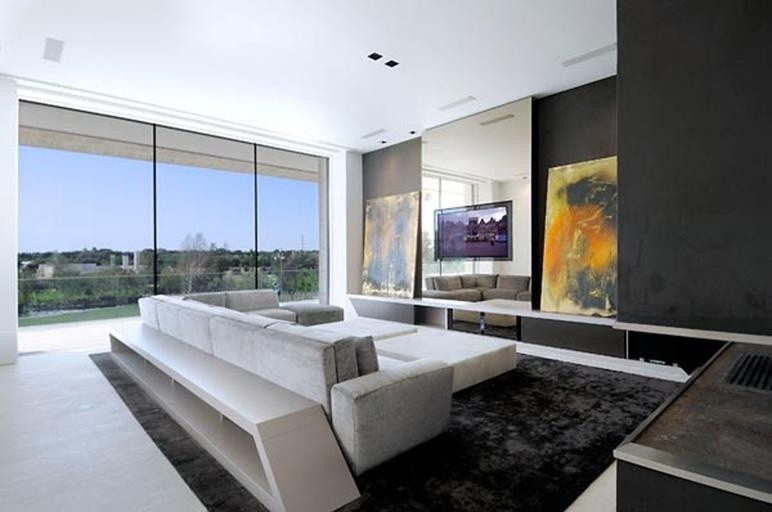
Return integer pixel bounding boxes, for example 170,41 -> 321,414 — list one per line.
85,341 -> 685,512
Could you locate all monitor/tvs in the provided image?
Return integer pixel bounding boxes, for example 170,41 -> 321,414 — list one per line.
433,199 -> 513,262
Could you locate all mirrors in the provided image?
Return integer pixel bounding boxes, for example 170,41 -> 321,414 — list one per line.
418,92 -> 539,302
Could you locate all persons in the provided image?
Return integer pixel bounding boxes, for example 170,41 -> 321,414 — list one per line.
272,282 -> 281,296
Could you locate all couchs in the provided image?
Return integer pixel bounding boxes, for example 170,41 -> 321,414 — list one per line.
421,273 -> 532,303
137,287 -> 449,486
